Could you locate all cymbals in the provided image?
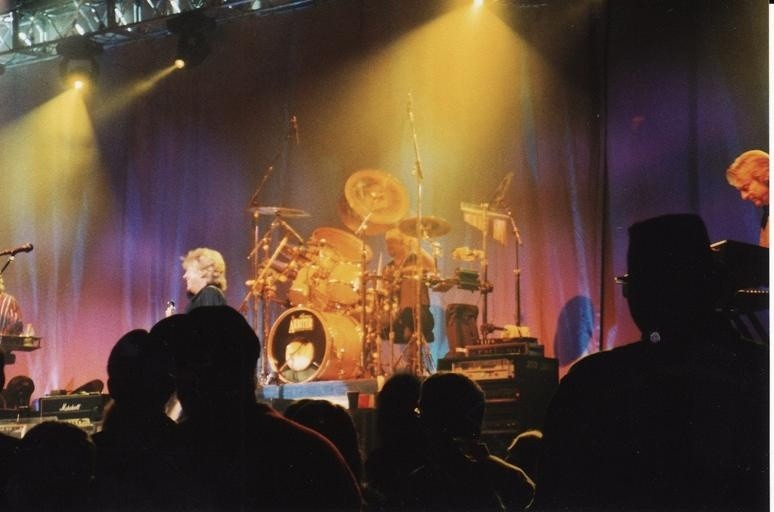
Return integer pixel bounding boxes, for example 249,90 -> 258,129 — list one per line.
453,249 -> 484,262
248,206 -> 309,219
281,246 -> 304,258
280,221 -> 303,243
399,217 -> 451,239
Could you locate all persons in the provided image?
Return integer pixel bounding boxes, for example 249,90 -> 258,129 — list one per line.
1,210 -> 769,511
725,149 -> 769,248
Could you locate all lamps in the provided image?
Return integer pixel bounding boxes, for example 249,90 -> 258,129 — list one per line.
56,9 -> 224,93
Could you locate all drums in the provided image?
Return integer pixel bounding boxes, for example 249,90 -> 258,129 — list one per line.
337,169 -> 410,236
326,261 -> 363,304
366,288 -> 398,321
289,265 -> 318,306
312,227 -> 374,262
266,307 -> 360,383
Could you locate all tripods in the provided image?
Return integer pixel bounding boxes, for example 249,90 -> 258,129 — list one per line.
391,184 -> 440,376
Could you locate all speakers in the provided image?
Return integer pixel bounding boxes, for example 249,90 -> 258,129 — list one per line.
39,392 -> 112,423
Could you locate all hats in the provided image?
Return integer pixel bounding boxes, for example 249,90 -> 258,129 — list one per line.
613,214 -> 712,287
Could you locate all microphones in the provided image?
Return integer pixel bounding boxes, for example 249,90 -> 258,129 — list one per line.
290,114 -> 301,146
170,300 -> 175,311
405,91 -> 416,116
0,241 -> 33,257
